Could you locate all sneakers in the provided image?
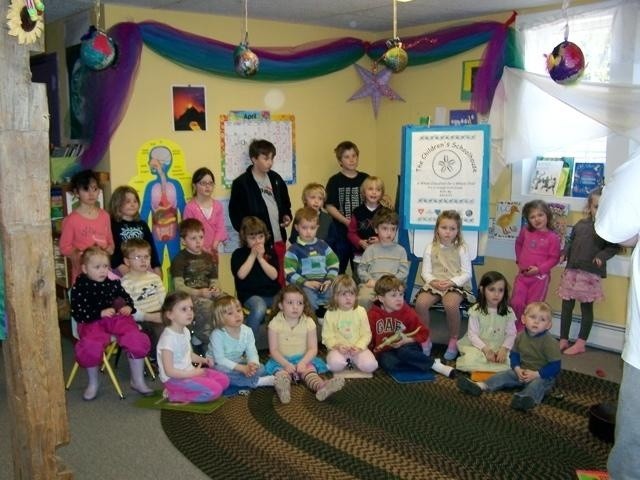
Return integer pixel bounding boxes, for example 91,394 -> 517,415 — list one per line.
558,339 -> 568,351
274,370 -> 292,404
563,337 -> 586,355
511,396 -> 535,410
457,375 -> 482,396
316,376 -> 345,402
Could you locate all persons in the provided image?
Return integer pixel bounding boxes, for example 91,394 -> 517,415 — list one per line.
108,185 -> 163,279
533,172 -> 558,196
183,168 -> 228,255
289,183 -> 339,247
58,168 -> 116,287
509,200 -> 561,332
229,140 -> 292,287
140,146 -> 186,267
323,140 -> 370,274
559,188 -> 621,355
69,211 -> 561,414
593,156 -> 640,480
347,175 -> 391,284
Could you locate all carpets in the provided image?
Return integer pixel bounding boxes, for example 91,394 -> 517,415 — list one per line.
159,342 -> 618,479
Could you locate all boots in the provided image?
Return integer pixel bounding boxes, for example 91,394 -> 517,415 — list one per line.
127,357 -> 154,396
83,366 -> 99,401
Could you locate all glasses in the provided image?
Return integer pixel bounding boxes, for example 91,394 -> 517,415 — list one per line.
197,181 -> 216,186
123,255 -> 151,261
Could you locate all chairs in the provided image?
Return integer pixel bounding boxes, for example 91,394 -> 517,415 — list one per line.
64,289 -> 155,401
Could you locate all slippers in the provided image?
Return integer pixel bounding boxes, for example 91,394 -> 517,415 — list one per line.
444,349 -> 459,361
422,343 -> 433,355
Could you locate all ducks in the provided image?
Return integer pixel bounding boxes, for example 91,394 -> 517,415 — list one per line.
496,206 -> 520,234
